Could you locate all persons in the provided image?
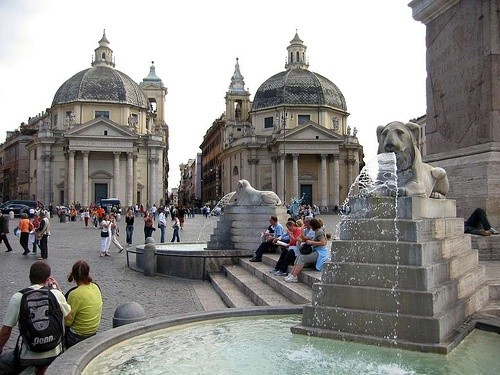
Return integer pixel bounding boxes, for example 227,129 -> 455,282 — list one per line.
18,213 -> 34,256
30,212 -> 52,259
64,260 -> 102,349
0,261 -> 72,375
151,204 -> 158,221
295,203 -> 321,217
159,205 -> 192,229
134,204 -> 145,217
124,210 -> 134,246
249,216 -> 330,282
100,205 -> 123,257
194,206 -> 210,219
170,215 -> 181,242
57,201 -> 81,223
157,209 -> 167,243
0,210 -> 12,252
143,210 -> 154,244
464,207 -> 497,236
34,202 -> 53,220
83,202 -> 105,229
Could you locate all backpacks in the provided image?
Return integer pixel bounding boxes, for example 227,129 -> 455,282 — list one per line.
19,286 -> 65,354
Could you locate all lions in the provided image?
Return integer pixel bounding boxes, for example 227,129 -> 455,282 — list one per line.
225,179 -> 282,206
357,121 -> 449,199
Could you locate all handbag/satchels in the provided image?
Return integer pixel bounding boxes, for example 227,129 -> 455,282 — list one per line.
101,231 -> 109,237
299,243 -> 313,255
14,227 -> 22,237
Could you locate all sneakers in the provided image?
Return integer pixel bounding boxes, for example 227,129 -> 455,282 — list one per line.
284,273 -> 299,282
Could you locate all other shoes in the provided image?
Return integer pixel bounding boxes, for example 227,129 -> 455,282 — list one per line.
250,258 -> 262,262
101,252 -> 103,257
22,250 -> 30,255
119,249 -> 123,253
5,249 -> 13,252
105,254 -> 109,256
270,269 -> 288,276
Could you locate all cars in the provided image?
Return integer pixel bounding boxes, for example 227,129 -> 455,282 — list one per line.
57,207 -> 70,217
0,203 -> 35,218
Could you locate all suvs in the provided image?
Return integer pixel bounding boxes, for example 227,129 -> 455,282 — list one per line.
0,200 -> 44,211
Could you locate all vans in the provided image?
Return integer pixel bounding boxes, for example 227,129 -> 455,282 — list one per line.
98,198 -> 122,217
